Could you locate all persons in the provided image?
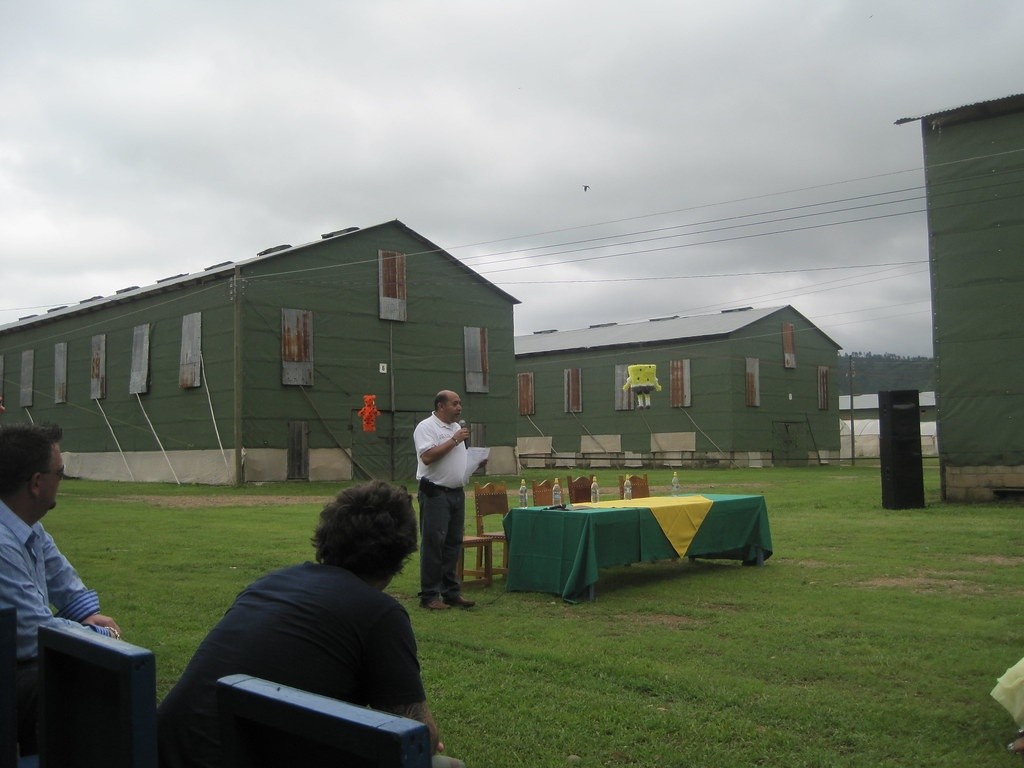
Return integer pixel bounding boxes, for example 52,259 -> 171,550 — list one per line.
157,481 -> 465,768
413,391 -> 488,610
0,421 -> 120,753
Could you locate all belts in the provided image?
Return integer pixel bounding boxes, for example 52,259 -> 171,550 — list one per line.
431,482 -> 462,493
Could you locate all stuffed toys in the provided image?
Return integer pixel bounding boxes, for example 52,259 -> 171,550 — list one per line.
623,365 -> 661,409
358,395 -> 381,432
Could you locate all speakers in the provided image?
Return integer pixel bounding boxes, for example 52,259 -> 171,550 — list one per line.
877,389 -> 925,510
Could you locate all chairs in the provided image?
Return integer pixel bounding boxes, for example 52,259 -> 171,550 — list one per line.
0,599 -> 434,768
456,534 -> 492,591
533,479 -> 564,506
619,473 -> 649,499
474,480 -> 508,579
567,475 -> 593,504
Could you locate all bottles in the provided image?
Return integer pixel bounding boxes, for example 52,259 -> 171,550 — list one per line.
624,473 -> 633,501
590,476 -> 599,503
672,472 -> 679,497
552,477 -> 562,507
519,478 -> 528,509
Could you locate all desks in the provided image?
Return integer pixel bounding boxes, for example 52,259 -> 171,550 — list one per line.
502,493 -> 773,604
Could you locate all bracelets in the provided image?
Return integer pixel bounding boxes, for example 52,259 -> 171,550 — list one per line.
105,626 -> 119,640
452,437 -> 458,445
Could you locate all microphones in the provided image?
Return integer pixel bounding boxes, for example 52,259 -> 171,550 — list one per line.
459,420 -> 468,450
543,503 -> 566,510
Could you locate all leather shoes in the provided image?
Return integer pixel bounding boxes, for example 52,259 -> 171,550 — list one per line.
444,596 -> 475,606
420,600 -> 451,609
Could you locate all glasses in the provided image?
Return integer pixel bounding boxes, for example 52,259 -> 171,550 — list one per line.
25,468 -> 65,480
